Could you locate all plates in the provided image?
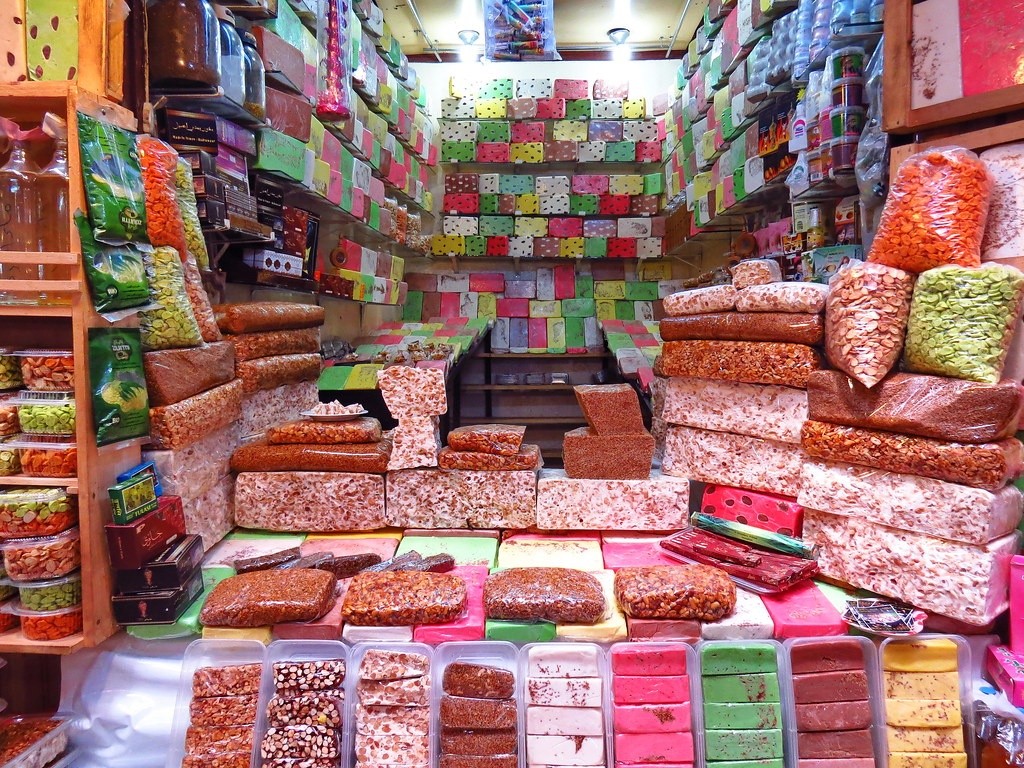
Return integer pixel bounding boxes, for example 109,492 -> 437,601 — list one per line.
840,594 -> 927,640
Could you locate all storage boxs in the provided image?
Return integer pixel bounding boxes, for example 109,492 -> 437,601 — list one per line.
103,459 -> 206,627
984,553 -> 1024,709
154,106 -> 321,297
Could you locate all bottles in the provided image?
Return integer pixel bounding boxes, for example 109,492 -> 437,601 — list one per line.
34,141 -> 70,306
0,139 -> 37,306
792,150 -> 807,184
793,104 -> 805,140
25,212 -> 27,213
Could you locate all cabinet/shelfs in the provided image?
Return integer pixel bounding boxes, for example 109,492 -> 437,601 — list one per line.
0,79 -> 139,656
452,346 -> 634,460
430,0 -> 1024,296
132,0 -> 441,312
